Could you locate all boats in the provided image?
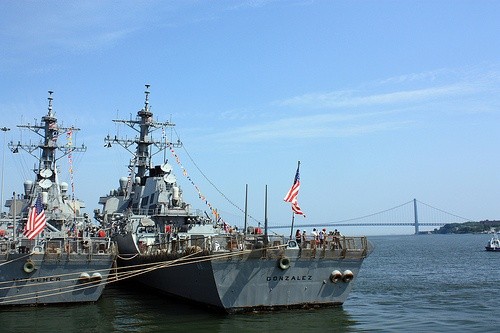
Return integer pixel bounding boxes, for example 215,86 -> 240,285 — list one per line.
0,89 -> 120,309
92,84 -> 375,315
485,232 -> 500,252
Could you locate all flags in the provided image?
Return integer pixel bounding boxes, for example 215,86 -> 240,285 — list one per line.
284,169 -> 306,218
22,192 -> 47,240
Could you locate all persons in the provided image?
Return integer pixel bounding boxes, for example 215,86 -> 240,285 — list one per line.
171,231 -> 178,253
228,222 -> 343,250
